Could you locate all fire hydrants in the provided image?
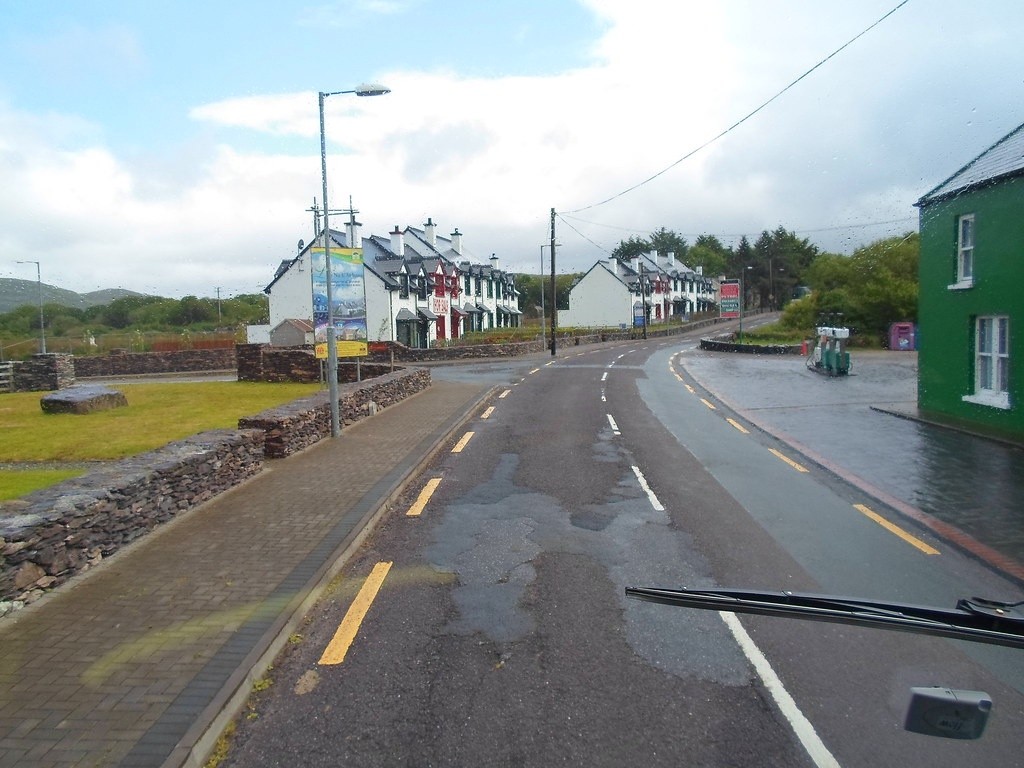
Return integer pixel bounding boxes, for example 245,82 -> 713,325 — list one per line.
801,340 -> 808,357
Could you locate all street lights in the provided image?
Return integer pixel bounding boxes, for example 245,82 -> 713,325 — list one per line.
17,261 -> 48,354
741,266 -> 752,318
319,80 -> 392,439
540,244 -> 563,353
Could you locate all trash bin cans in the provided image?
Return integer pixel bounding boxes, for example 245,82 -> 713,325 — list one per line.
888,321 -> 914,351
803,340 -> 812,356
